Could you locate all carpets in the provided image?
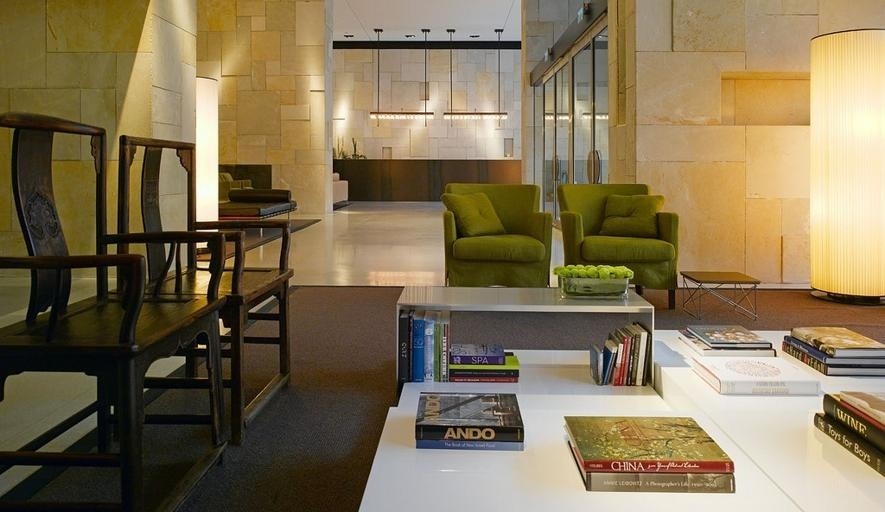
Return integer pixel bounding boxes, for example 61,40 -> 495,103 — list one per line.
0,284 -> 884,508
196,218 -> 322,261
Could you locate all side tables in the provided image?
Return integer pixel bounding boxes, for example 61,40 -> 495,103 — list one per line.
679,270 -> 761,322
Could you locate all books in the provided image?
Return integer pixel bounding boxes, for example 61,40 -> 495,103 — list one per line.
812,390 -> 885,477
675,324 -> 885,395
562,414 -> 735,492
584,323 -> 652,386
398,308 -> 522,383
414,391 -> 525,453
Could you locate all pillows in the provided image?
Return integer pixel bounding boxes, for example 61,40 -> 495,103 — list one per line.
598,194 -> 666,241
440,191 -> 504,238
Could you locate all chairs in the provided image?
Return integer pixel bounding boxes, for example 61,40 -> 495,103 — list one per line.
106,134 -> 295,449
555,183 -> 680,310
0,110 -> 223,511
440,183 -> 555,289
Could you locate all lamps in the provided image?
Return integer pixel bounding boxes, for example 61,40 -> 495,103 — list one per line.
369,28 -> 508,126
